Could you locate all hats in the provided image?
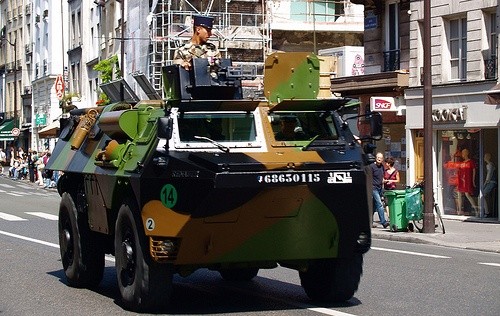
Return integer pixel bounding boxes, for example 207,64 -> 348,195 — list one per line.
192,15 -> 215,29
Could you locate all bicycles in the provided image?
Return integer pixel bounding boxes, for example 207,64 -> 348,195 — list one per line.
413,180 -> 445,233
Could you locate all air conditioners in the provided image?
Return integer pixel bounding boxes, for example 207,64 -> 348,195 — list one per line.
318,46 -> 364,78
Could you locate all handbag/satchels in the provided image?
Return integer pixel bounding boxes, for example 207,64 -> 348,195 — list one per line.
383,182 -> 391,190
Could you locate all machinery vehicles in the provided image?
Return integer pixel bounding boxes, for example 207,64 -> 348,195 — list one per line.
43,51 -> 373,310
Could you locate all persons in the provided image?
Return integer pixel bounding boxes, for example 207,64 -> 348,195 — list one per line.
444,147 -> 460,215
456,148 -> 480,217
477,151 -> 497,218
0,145 -> 63,189
274,113 -> 326,141
383,158 -> 400,190
370,153 -> 390,228
173,14 -> 223,71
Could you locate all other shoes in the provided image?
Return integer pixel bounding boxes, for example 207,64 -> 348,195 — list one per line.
44,186 -> 47,189
39,184 -> 43,186
372,225 -> 377,228
11,177 -> 18,179
22,177 -> 24,180
383,223 -> 390,228
